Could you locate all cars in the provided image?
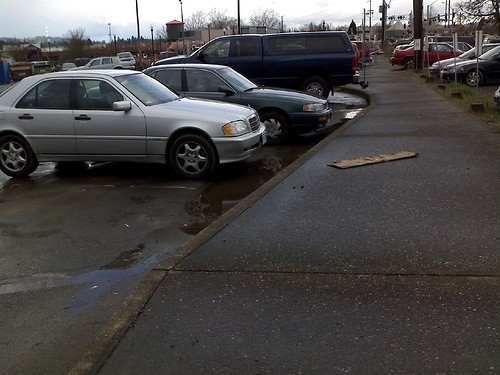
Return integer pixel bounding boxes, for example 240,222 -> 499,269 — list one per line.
60,63 -> 76,71
86,52 -> 137,68
430,42 -> 500,103
389,35 -> 500,71
86,63 -> 334,144
0,67 -> 270,181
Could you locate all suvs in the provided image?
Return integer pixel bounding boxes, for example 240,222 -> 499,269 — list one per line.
148,31 -> 362,100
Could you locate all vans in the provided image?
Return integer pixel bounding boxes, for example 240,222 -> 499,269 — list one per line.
351,40 -> 372,63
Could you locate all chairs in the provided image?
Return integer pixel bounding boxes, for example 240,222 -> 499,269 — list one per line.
99,81 -> 120,110
207,75 -> 219,91
